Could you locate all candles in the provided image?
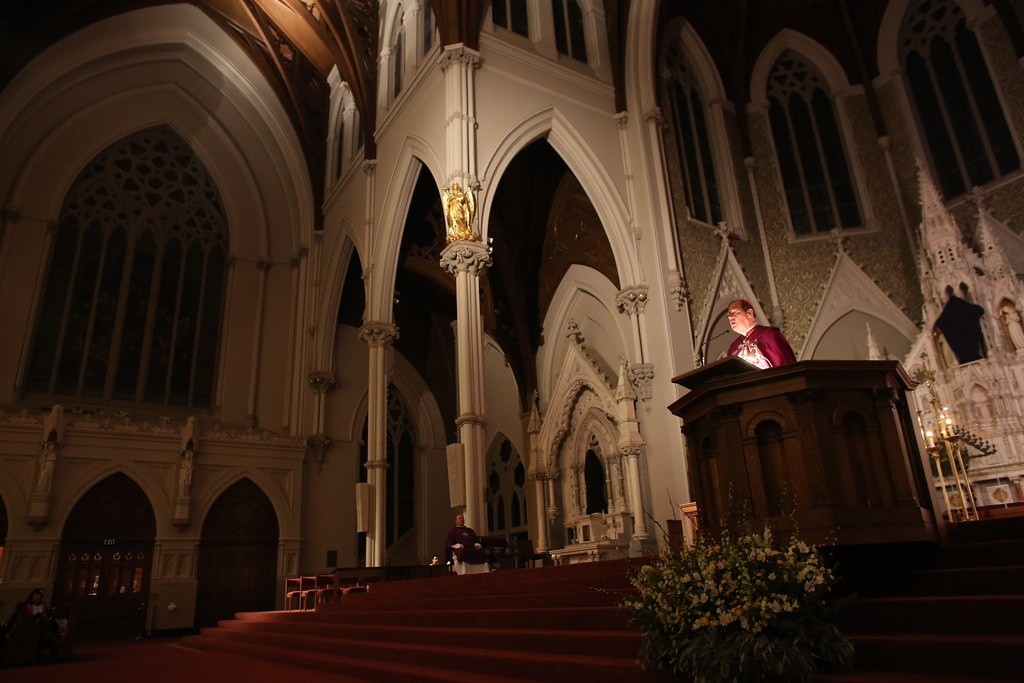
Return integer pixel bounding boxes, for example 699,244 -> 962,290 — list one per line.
924,405 -> 955,446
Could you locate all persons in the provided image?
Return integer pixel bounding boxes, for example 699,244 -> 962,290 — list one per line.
727,298 -> 797,368
23,590 -> 47,618
451,514 -> 490,573
429,555 -> 439,566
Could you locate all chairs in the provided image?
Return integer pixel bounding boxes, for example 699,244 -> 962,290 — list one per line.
284,578 -> 308,611
299,576 -> 326,611
339,568 -> 366,595
450,534 -> 546,571
314,574 -> 339,609
